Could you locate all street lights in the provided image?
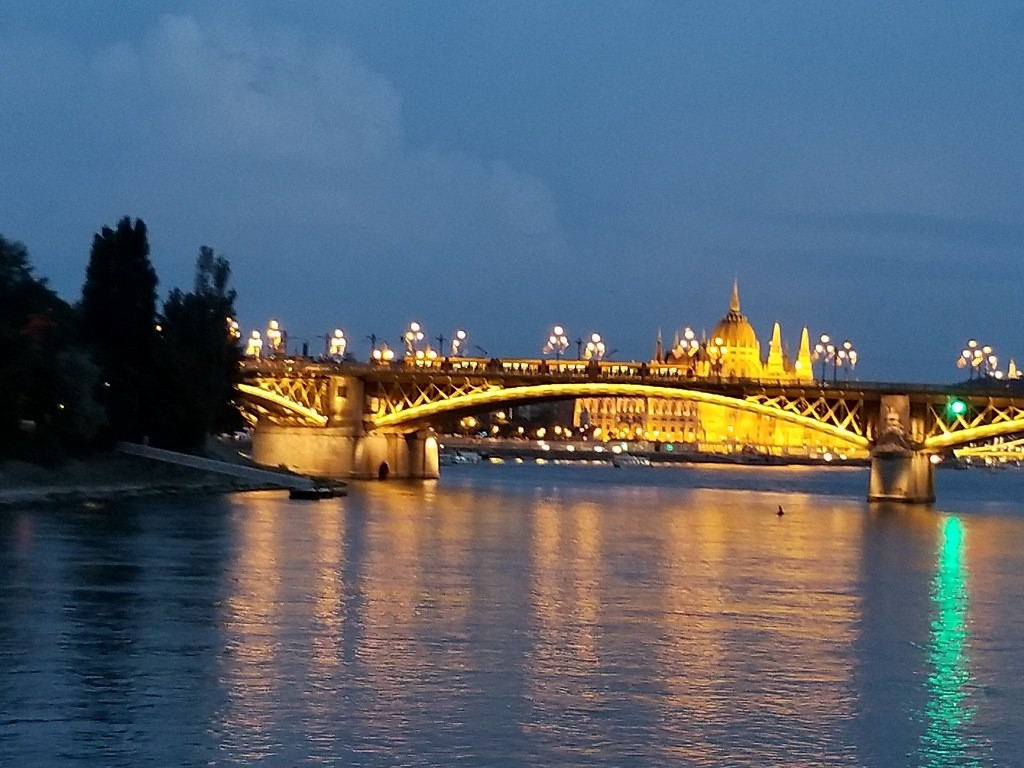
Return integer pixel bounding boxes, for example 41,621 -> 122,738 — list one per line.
812,334 -> 858,383
956,339 -> 994,379
